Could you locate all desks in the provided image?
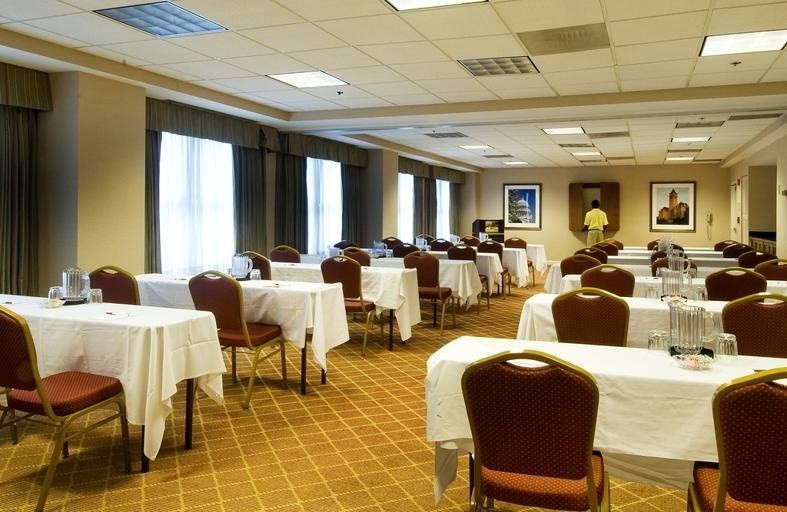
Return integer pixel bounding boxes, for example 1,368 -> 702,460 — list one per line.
133,273 -> 350,395
486,242 -> 548,287
516,292 -> 782,349
617,250 -> 722,258
623,246 -> 713,250
269,262 -> 421,351
544,263 -> 754,294
424,335 -> 787,507
424,251 -> 503,309
369,258 -> 482,327
0,293 -> 225,472
607,255 -> 737,267
557,274 -> 786,301
456,246 -> 529,295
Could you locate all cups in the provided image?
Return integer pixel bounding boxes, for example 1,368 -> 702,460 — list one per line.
648,330 -> 671,362
667,249 -> 684,257
714,333 -> 738,361
233,256 -> 253,278
668,257 -> 691,272
479,232 -> 488,243
669,305 -> 719,353
416,236 -> 427,249
63,264 -> 90,298
450,235 -> 460,245
661,271 -> 692,295
656,267 -> 667,280
374,243 -> 387,258
328,248 -> 344,261
687,268 -> 697,279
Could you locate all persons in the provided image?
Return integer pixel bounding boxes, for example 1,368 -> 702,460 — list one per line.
581,199 -> 609,248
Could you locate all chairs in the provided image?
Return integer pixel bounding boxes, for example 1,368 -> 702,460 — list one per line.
413,234 -> 434,245
550,287 -> 630,347
504,237 -> 535,286
753,258 -> 786,281
459,236 -> 480,246
704,267 -> 767,302
650,250 -> 687,265
342,247 -> 370,266
188,270 -> 287,408
320,255 -> 384,355
560,254 -> 601,278
270,245 -> 300,263
652,243 -> 683,253
88,265 -> 140,305
392,243 -> 420,258
460,347 -> 610,511
722,242 -> 754,258
476,240 -> 510,296
713,240 -> 738,251
646,239 -> 660,250
737,250 -> 777,268
651,257 -> 696,277
591,242 -> 618,256
580,264 -> 635,297
380,236 -> 403,249
430,238 -> 453,251
721,292 -> 786,358
333,240 -> 357,250
573,247 -> 607,264
599,238 -> 623,250
447,244 -> 489,314
0,304 -> 132,512
686,366 -> 787,511
403,251 -> 455,336
231,251 -> 271,281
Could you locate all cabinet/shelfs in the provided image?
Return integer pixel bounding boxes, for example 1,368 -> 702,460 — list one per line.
568,182 -> 619,232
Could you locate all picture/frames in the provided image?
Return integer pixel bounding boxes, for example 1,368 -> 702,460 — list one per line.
648,181 -> 696,233
502,182 -> 542,231
